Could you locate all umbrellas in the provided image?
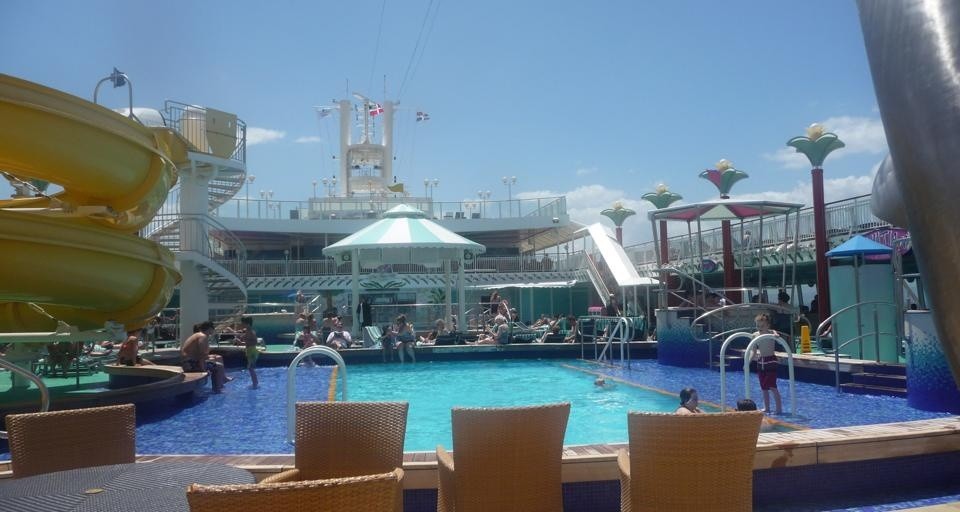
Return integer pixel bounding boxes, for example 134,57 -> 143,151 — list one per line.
824,232 -> 895,359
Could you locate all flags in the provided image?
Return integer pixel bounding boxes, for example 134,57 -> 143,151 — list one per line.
368,103 -> 384,117
319,109 -> 331,117
416,112 -> 430,121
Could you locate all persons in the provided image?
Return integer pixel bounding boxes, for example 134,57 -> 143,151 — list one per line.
743,314 -> 784,416
735,399 -> 774,433
72,315 -> 259,396
672,388 -> 706,415
292,288 -> 820,369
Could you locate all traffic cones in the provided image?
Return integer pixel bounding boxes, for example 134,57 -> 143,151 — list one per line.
800,325 -> 811,354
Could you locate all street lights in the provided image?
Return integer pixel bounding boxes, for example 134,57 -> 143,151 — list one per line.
600,201 -> 636,247
245,176 -> 516,218
697,159 -> 749,304
786,122 -> 845,328
640,183 -> 682,307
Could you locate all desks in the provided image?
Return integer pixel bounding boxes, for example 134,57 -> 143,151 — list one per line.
0,462 -> 255,512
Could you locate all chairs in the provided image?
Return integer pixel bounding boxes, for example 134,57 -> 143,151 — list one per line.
617,410 -> 763,512
360,315 -> 646,348
435,401 -> 570,512
5,403 -> 136,479
257,401 -> 409,511
185,472 -> 399,512
